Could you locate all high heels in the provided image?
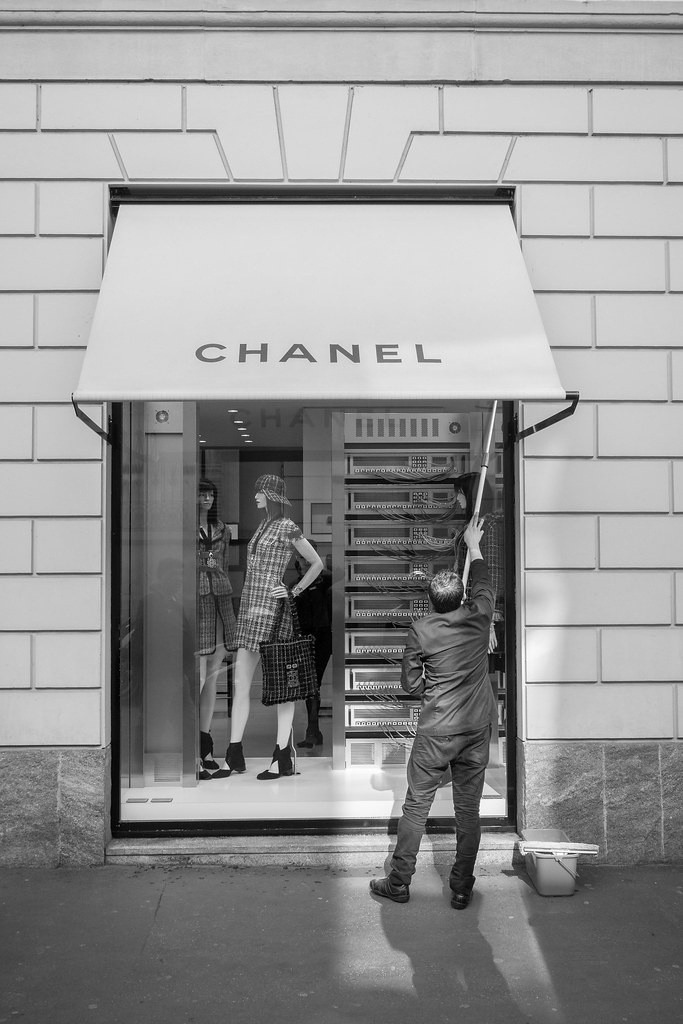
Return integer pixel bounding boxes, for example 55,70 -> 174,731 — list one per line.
256,743 -> 293,780
200,731 -> 219,770
199,770 -> 212,780
212,742 -> 246,779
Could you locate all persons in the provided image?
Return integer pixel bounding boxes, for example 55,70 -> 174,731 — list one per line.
434,469 -> 503,789
196,476 -> 323,779
289,540 -> 332,749
369,509 -> 496,910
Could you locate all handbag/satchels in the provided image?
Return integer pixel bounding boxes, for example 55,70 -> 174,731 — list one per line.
259,591 -> 318,707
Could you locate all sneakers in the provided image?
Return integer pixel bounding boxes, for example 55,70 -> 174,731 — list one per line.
451,890 -> 473,909
370,876 -> 410,903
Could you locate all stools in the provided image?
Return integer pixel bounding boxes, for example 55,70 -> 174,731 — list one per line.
216,654 -> 234,717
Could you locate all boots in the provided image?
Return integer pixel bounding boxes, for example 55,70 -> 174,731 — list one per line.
297,699 -> 323,748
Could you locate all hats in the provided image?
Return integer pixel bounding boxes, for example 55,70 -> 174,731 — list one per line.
254,475 -> 292,507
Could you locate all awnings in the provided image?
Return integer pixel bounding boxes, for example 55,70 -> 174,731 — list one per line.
69,195 -> 585,446
